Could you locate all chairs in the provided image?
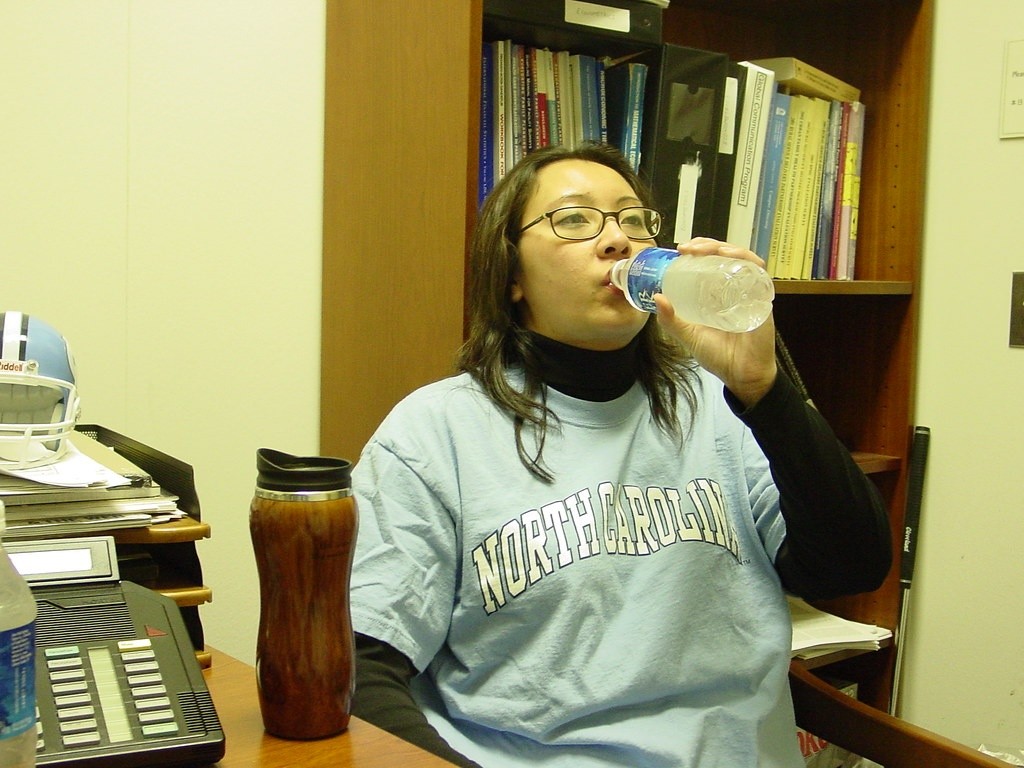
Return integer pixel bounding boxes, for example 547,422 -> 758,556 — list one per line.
787,664 -> 1024,768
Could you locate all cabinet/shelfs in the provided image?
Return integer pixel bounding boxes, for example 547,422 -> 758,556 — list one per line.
319,0 -> 936,714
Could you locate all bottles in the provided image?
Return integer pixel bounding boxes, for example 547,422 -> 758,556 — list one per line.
0,499 -> 37,768
610,246 -> 774,333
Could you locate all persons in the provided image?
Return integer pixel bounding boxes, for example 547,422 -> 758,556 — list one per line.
350,142 -> 896,766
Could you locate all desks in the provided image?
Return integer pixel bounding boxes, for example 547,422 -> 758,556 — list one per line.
203,645 -> 461,768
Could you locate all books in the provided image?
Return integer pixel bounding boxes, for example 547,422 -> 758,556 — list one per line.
479,38 -> 868,282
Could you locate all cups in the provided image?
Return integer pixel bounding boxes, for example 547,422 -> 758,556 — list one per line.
249,447 -> 361,739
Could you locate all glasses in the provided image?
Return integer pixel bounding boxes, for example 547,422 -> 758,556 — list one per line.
513,205 -> 666,247
